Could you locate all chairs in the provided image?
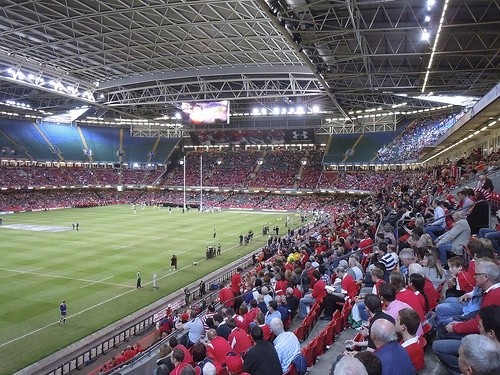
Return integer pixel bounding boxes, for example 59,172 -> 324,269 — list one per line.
288,293 -> 354,375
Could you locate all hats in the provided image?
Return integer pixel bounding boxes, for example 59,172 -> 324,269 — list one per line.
182,314 -> 188,319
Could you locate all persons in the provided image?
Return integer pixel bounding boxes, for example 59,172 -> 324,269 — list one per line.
0,112 -> 500,375
183,103 -> 208,124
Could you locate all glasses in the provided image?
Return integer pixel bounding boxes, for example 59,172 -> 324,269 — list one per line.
475,273 -> 485,276
